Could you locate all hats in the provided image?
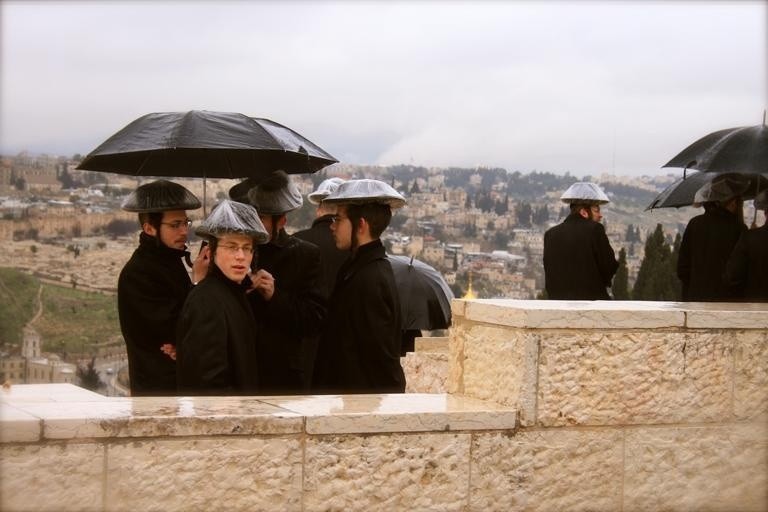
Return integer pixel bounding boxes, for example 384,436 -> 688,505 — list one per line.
121,182 -> 202,212
321,179 -> 406,209
307,178 -> 345,206
695,178 -> 751,203
753,189 -> 768,210
228,173 -> 303,214
560,182 -> 610,204
195,200 -> 269,244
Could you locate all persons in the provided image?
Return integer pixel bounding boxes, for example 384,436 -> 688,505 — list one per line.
290,177 -> 353,306
176,201 -> 276,397
540,181 -> 621,300
675,174 -> 752,304
230,176 -> 328,394
724,189 -> 767,300
320,177 -> 407,392
116,178 -> 214,397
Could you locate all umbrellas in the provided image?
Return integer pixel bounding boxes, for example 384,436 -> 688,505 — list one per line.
661,111 -> 768,227
641,169 -> 767,212
386,253 -> 457,332
75,109 -> 340,221
